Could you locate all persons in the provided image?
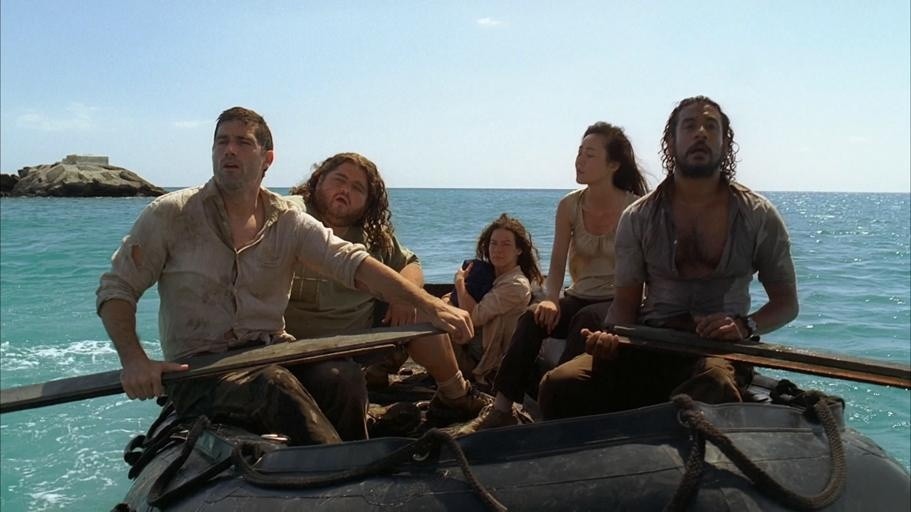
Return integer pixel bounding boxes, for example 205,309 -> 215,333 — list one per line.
96,106 -> 476,446
459,122 -> 650,437
536,98 -> 798,421
441,213 -> 543,386
285,154 -> 496,441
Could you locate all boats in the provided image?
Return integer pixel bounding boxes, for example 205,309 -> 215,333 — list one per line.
116,281 -> 911,511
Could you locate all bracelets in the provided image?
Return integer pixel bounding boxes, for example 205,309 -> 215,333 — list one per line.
736,312 -> 760,341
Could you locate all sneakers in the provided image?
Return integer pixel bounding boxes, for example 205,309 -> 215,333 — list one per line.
456,401 -> 518,435
426,380 -> 495,419
